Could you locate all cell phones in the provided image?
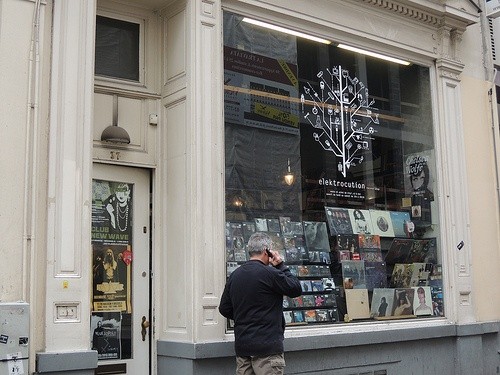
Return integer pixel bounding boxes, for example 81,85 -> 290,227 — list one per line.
266,249 -> 273,258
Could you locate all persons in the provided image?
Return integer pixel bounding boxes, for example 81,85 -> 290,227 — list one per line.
218,232 -> 302,375
